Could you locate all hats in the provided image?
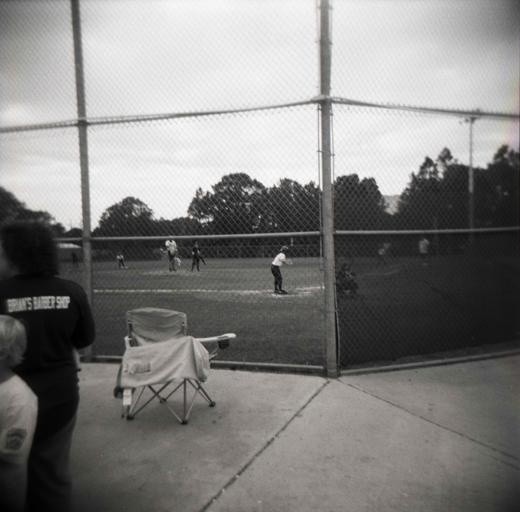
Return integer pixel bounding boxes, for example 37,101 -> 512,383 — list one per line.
280,246 -> 288,251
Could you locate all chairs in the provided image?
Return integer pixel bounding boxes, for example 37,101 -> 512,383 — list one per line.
121,307 -> 238,423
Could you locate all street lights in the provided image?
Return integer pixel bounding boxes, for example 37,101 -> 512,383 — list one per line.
458,106 -> 482,240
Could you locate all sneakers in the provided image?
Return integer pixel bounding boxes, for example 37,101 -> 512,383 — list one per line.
274,290 -> 288,294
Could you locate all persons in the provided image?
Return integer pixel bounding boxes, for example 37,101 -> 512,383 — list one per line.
190,240 -> 201,272
418,234 -> 431,261
0,313 -> 41,512
159,239 -> 178,272
0,220 -> 96,511
116,251 -> 125,269
335,248 -> 359,299
269,245 -> 293,294
